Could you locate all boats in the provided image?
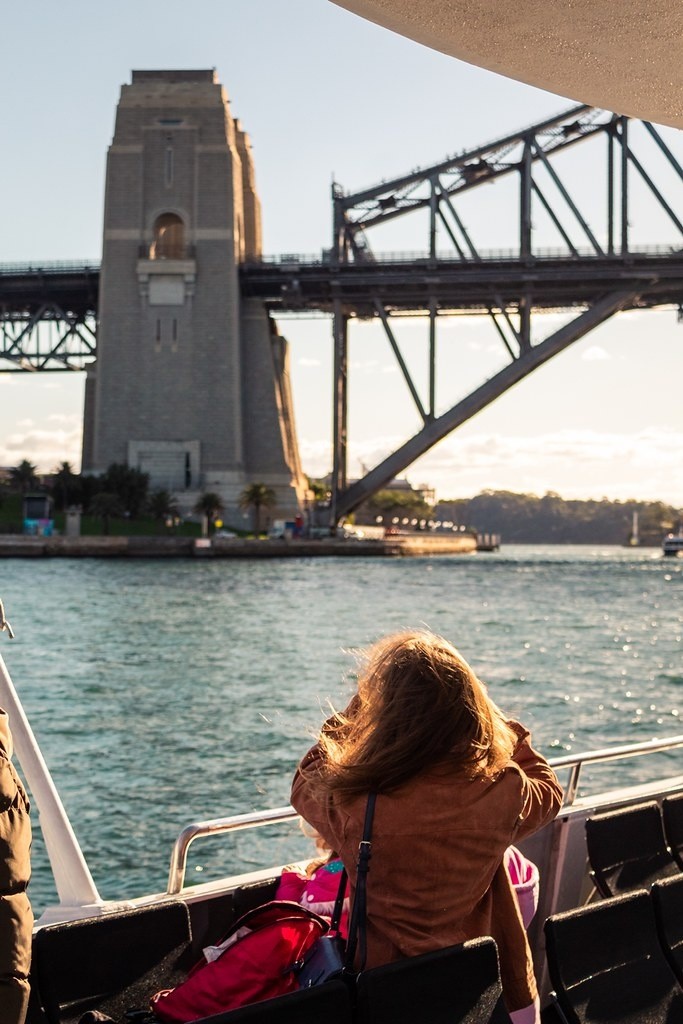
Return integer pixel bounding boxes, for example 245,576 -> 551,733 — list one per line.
662,537 -> 683,557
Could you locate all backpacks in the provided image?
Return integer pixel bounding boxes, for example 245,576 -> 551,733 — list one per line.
150,900 -> 331,1022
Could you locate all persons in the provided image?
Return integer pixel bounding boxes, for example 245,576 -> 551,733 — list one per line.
0,599 -> 35,1023
290,628 -> 565,1023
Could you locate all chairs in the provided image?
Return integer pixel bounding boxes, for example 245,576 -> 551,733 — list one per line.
189,790 -> 683,1024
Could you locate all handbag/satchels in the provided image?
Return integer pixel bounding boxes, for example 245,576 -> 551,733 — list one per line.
357,936 -> 513,1024
294,937 -> 345,1003
